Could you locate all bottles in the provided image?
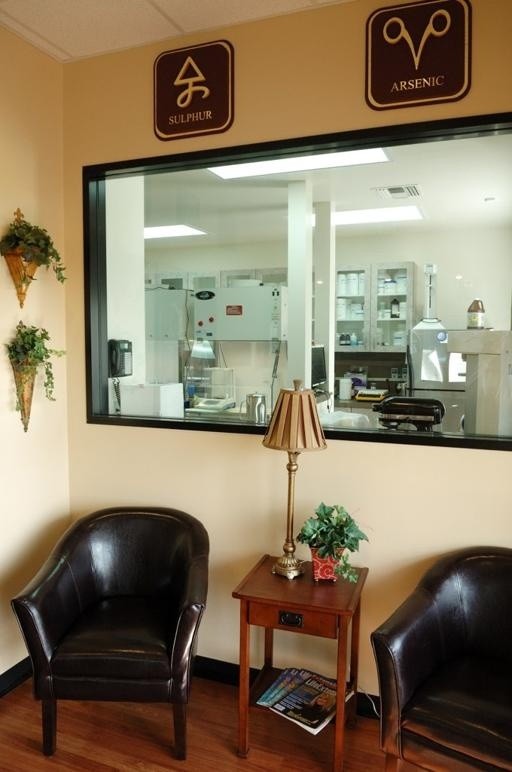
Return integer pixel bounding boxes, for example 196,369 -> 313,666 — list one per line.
340,332 -> 357,346
390,297 -> 400,319
239,392 -> 266,424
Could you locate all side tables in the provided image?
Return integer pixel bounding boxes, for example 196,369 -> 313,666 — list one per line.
232,553 -> 370,771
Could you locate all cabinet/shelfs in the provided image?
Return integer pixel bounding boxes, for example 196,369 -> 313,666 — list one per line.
156,260 -> 430,354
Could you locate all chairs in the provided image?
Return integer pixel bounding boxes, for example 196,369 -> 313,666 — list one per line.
369,546 -> 511,771
10,505 -> 209,762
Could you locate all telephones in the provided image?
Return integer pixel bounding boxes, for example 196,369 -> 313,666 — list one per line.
108,339 -> 132,377
355,388 -> 389,401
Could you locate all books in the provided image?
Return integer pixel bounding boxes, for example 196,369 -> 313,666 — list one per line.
255,667 -> 355,736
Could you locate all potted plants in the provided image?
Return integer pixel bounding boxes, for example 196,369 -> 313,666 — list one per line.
2,320 -> 66,432
1,219 -> 68,310
296,501 -> 369,584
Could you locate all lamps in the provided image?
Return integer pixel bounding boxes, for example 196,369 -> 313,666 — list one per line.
262,379 -> 328,582
